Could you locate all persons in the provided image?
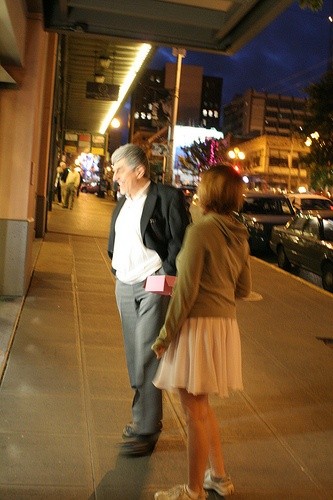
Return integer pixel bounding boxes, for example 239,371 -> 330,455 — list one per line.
108,144 -> 193,455
152,165 -> 253,500
55,162 -> 119,210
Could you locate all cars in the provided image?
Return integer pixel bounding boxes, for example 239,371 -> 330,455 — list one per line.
80,169 -> 124,202
177,184 -> 197,211
269,212 -> 333,293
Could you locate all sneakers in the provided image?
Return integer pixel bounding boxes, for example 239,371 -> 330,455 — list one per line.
154,485 -> 206,500
203,469 -> 234,495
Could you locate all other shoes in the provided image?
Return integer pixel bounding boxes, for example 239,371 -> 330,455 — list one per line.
124,421 -> 161,438
120,431 -> 160,457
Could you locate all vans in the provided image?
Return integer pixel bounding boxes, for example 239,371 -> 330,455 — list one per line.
282,193 -> 333,221
235,192 -> 296,258
